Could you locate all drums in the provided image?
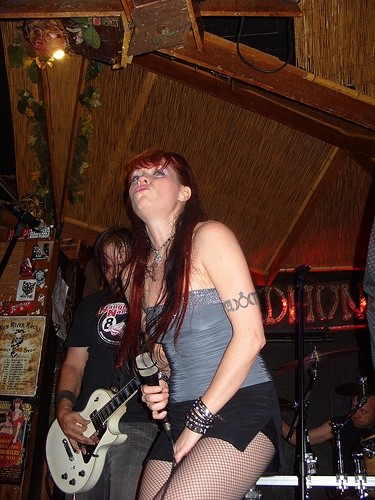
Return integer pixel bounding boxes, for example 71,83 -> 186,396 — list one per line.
354,432 -> 375,497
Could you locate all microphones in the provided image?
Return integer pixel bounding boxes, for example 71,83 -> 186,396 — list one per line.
135,352 -> 174,441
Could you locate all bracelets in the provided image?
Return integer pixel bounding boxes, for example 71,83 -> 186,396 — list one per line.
183,397 -> 215,435
55,390 -> 76,408
159,375 -> 169,384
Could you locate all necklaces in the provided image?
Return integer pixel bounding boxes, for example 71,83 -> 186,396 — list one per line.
151,234 -> 174,261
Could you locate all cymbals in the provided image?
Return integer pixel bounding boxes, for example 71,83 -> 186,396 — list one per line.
276,347 -> 360,370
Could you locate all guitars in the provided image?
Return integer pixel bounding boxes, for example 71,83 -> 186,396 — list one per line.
45,375 -> 144,494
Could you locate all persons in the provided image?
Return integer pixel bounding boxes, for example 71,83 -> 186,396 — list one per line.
281,395 -> 375,500
114,148 -> 282,500
54,226 -> 162,500
